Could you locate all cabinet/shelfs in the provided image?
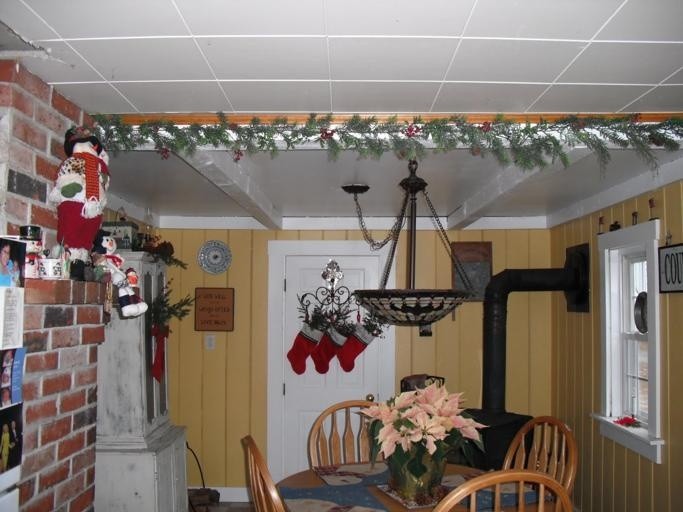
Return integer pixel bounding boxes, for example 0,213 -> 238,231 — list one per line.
96,252 -> 190,512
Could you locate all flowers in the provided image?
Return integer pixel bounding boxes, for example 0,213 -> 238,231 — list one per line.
358,380 -> 489,468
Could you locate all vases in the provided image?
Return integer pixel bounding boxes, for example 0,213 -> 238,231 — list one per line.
399,453 -> 432,502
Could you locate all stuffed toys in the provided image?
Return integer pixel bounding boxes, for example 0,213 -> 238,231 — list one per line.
124,267 -> 138,288
88,228 -> 149,318
49,125 -> 112,284
17,224 -> 49,280
90,252 -> 113,284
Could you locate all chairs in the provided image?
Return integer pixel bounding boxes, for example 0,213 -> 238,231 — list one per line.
308,401 -> 379,462
432,470 -> 575,509
500,416 -> 576,511
240,435 -> 289,511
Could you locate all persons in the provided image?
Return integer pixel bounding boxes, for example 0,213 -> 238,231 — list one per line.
7,419 -> 23,467
0,367 -> 12,388
0,239 -> 22,287
2,350 -> 14,367
9,258 -> 20,287
0,388 -> 12,407
0,423 -> 12,472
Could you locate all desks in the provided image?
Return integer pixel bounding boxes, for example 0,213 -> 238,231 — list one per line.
275,462 -> 555,511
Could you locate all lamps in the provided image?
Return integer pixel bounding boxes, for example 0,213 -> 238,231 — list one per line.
341,155 -> 478,327
